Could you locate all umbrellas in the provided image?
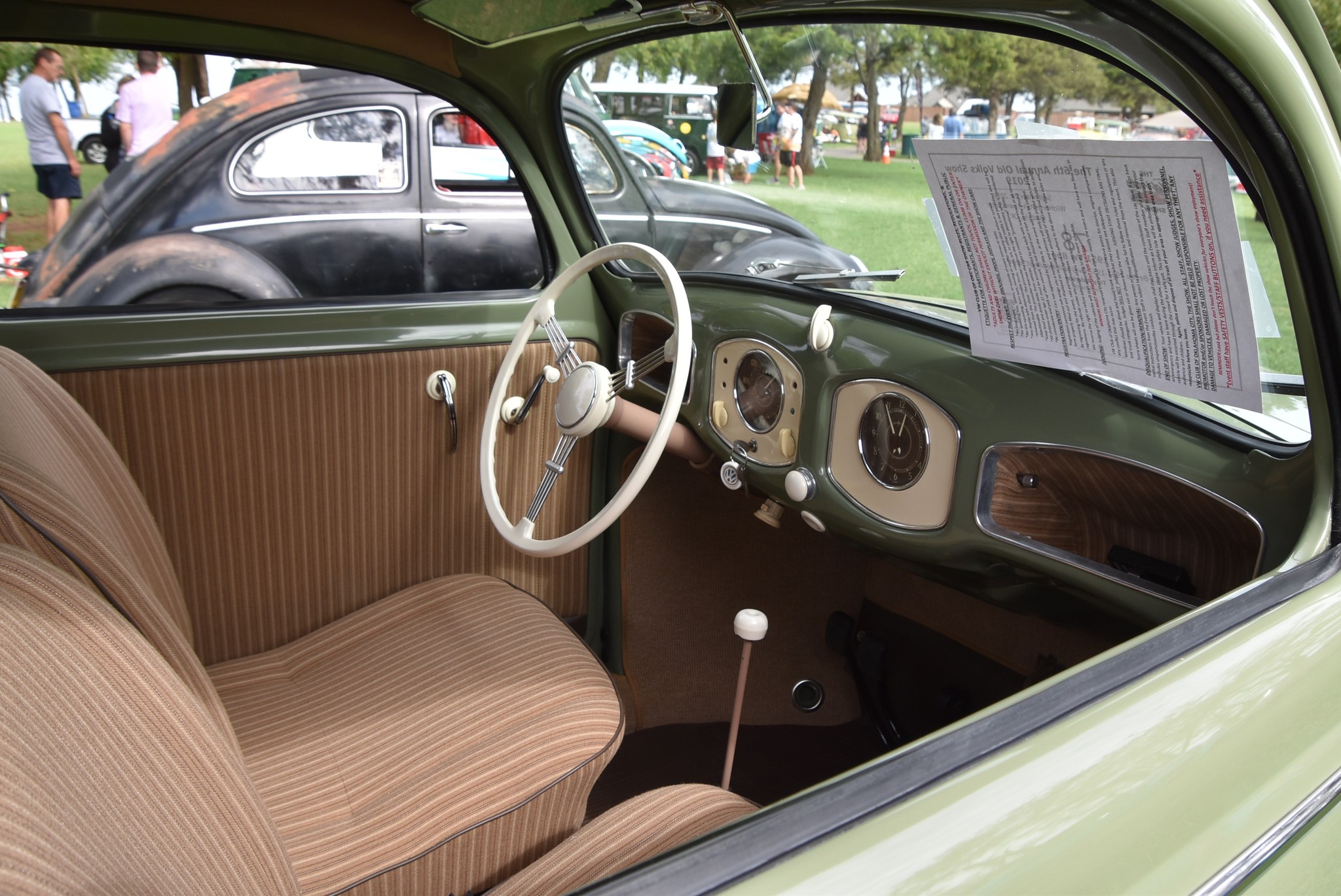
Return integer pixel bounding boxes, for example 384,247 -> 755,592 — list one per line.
771,84 -> 845,111
1137,110 -> 1199,129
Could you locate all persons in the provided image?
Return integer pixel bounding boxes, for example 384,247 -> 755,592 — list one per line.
458,114 -> 497,146
944,107 -> 964,139
19,47 -> 85,244
705,109 -> 727,186
767,101 -> 795,185
101,74 -> 136,173
112,49 -> 178,160
434,113 -> 461,145
822,115 -> 883,153
927,112 -> 944,140
725,146 -> 761,186
766,102 -> 806,190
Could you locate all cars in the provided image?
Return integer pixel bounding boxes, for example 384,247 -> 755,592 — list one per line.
61,118 -> 182,165
7,65 -> 907,308
0,3 -> 1340,895
224,67 -> 693,192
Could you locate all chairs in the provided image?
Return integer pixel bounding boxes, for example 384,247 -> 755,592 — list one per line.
0,542 -> 765,896
758,132 -> 774,163
812,135 -> 828,168
0,343 -> 625,895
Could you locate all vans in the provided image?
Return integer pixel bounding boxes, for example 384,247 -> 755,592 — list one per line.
587,82 -> 718,179
